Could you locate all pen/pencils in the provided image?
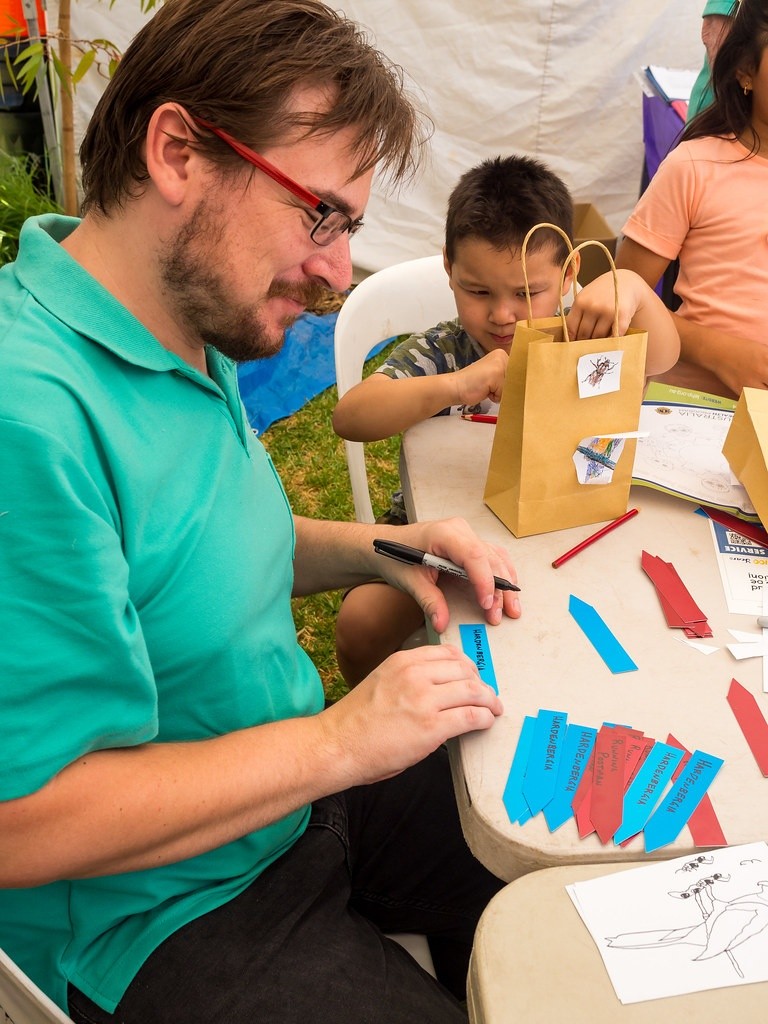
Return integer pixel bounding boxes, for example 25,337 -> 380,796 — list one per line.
461,414 -> 497,425
552,505 -> 642,569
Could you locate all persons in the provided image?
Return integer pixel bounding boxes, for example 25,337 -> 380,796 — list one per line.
613,0 -> 767,394
333,153 -> 680,693
657,0 -> 748,308
1,0 -> 535,1018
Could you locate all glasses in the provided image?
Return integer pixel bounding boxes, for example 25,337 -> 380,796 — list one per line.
189,112 -> 365,247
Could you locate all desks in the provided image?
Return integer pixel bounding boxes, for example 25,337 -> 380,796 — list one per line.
399,412 -> 768,887
468,863 -> 768,1022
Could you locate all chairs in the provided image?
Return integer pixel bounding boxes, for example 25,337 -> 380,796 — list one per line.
335,256 -> 583,523
0,936 -> 437,1024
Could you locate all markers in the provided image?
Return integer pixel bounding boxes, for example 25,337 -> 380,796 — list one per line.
371,538 -> 522,596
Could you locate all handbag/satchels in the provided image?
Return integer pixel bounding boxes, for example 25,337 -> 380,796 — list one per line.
480,221 -> 650,540
